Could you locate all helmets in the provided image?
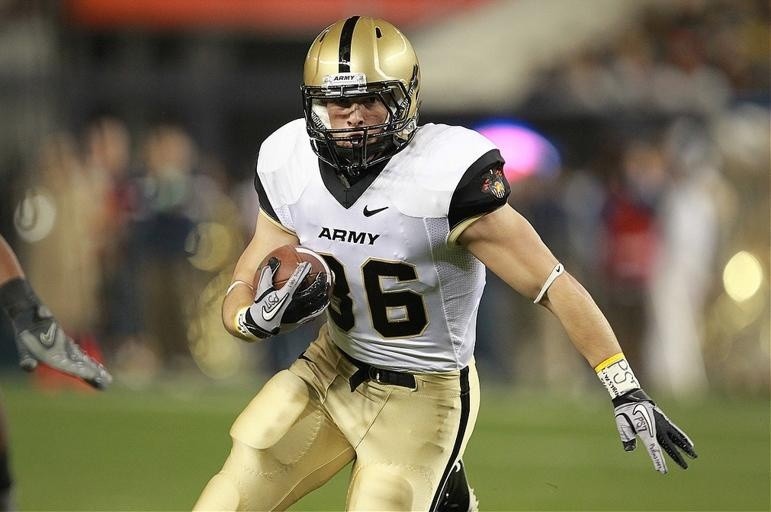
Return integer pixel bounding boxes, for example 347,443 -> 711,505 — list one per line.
302,15 -> 419,134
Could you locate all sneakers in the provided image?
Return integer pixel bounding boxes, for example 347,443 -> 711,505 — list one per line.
437,459 -> 479,512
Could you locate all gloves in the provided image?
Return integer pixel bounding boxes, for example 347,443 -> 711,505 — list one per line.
234,257 -> 331,343
0,276 -> 113,392
593,351 -> 698,475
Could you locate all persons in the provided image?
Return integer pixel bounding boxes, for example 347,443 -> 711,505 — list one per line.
187,17 -> 698,511
0,236 -> 114,393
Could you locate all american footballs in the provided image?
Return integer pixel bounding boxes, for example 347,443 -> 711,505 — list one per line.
252,244 -> 334,307
